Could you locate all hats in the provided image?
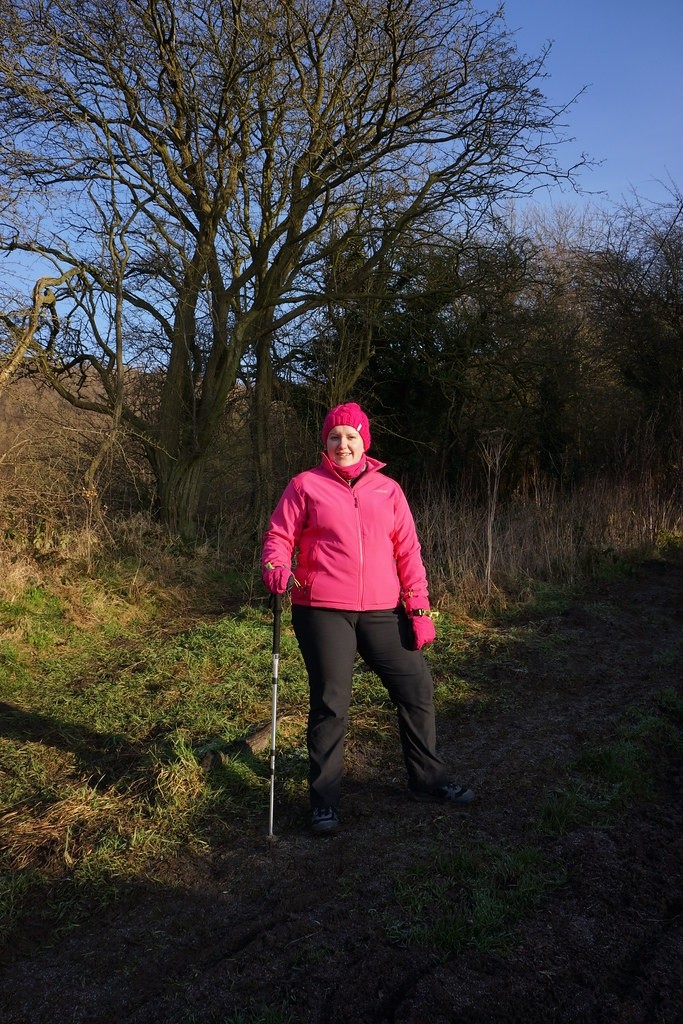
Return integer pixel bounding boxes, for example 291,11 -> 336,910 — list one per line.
322,401 -> 371,453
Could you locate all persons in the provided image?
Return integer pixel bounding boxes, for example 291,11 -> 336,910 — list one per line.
263,402 -> 476,833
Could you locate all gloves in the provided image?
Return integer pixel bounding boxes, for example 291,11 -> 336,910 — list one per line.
263,565 -> 295,595
412,616 -> 436,650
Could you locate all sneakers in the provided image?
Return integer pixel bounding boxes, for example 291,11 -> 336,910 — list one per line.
413,783 -> 476,804
312,807 -> 340,835
